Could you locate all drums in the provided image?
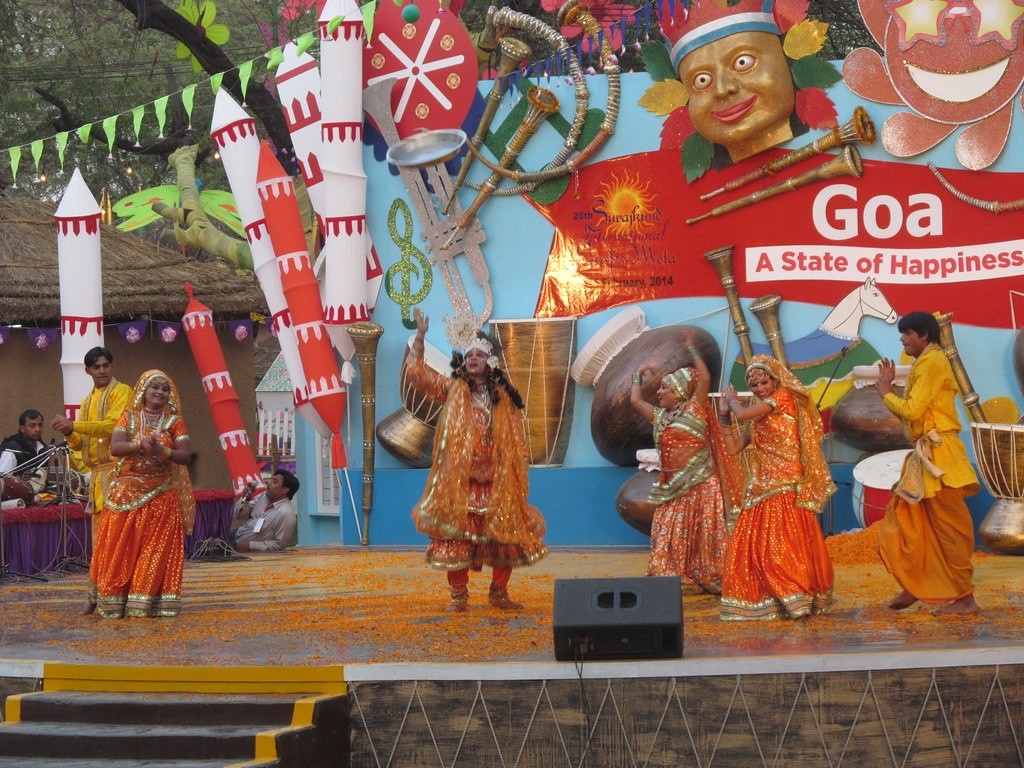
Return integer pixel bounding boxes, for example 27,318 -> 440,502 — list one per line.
0,472 -> 36,508
30,465 -> 85,497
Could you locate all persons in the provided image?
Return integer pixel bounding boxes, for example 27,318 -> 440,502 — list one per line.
655,0 -> 795,165
719,353 -> 835,621
879,310 -> 981,614
0,409 -> 49,477
631,329 -> 744,595
50,345 -> 133,615
407,307 -> 548,609
231,470 -> 300,552
90,371 -> 196,618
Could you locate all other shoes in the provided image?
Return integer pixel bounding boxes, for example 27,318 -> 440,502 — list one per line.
489,590 -> 523,610
447,596 -> 467,613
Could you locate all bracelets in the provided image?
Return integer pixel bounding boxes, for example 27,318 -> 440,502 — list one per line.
720,416 -> 734,436
160,448 -> 172,459
415,338 -> 424,344
130,440 -> 142,452
688,344 -> 699,361
632,372 -> 642,386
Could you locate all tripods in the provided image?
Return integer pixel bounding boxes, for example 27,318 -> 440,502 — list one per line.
0,442 -> 90,582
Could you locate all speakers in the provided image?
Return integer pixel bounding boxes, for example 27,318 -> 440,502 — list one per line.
553,576 -> 685,660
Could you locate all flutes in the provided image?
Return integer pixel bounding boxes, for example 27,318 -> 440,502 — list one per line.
703,242 -> 792,371
935,311 -> 989,424
439,36 -> 561,251
344,320 -> 386,546
685,105 -> 877,226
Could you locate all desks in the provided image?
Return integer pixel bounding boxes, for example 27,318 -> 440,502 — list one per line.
0,488 -> 234,577
